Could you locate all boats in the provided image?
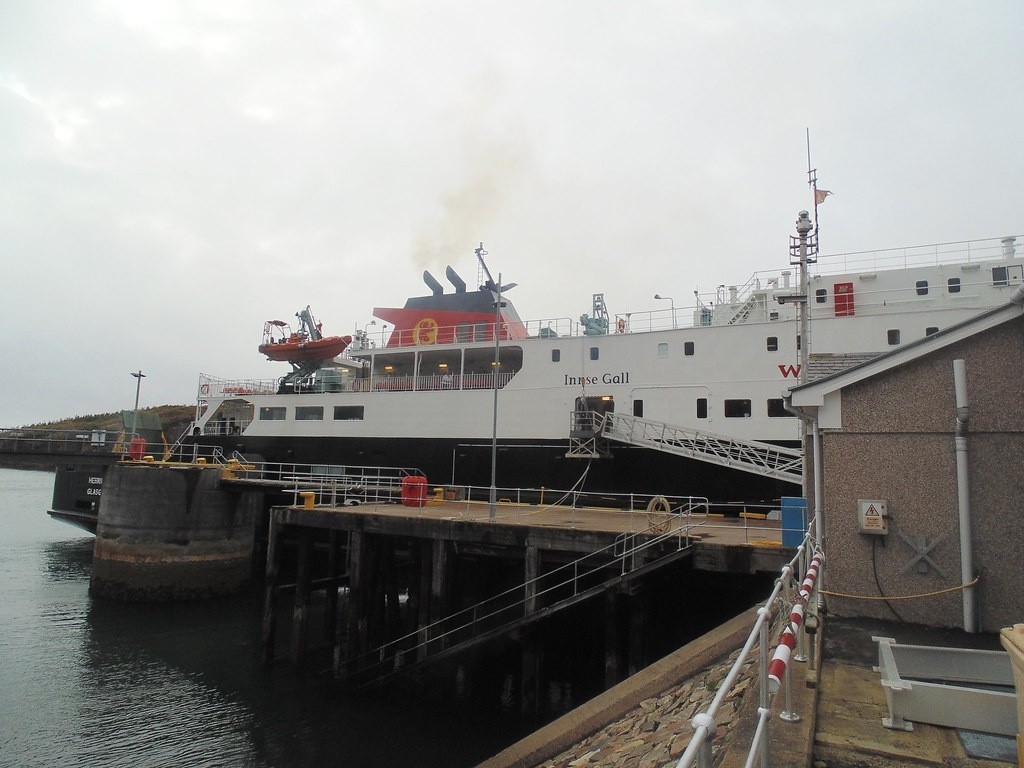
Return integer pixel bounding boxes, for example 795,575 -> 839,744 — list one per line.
45,236 -> 1023,539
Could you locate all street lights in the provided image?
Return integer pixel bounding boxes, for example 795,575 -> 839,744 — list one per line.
130,369 -> 146,458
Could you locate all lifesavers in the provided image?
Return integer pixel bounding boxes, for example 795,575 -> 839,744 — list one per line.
201,384 -> 209,394
619,319 -> 624,333
